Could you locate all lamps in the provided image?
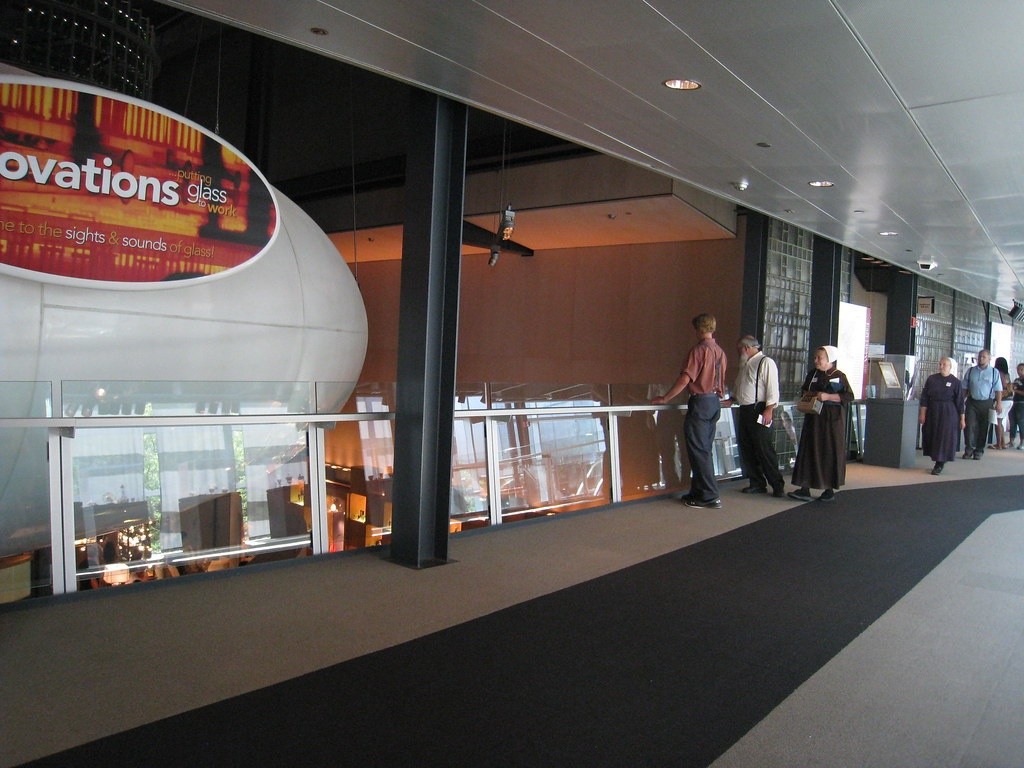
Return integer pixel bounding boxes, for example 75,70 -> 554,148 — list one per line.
64,397 -> 240,418
103,563 -> 129,583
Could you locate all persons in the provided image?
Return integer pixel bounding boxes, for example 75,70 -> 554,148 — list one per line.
963,350 -> 1003,460
720,336 -> 785,497
1005,364 -> 1024,449
919,357 -> 965,475
989,357 -> 1014,450
652,314 -> 727,508
787,346 -> 854,501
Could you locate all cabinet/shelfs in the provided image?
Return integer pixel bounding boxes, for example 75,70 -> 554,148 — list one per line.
864,397 -> 919,467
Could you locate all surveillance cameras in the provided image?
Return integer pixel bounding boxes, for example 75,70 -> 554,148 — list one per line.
919,263 -> 937,270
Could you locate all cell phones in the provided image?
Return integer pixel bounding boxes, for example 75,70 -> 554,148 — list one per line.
757,414 -> 772,428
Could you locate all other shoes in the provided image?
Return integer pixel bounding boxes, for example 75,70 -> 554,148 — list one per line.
685,497 -> 721,509
1006,442 -> 1014,449
743,487 -> 767,494
773,488 -> 784,497
788,489 -> 811,501
931,463 -> 944,475
820,489 -> 835,502
681,494 -> 693,503
974,453 -> 980,459
987,444 -> 1006,449
1017,442 -> 1024,450
962,454 -> 971,460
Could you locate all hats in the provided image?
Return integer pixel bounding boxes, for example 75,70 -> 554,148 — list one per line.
822,345 -> 840,363
